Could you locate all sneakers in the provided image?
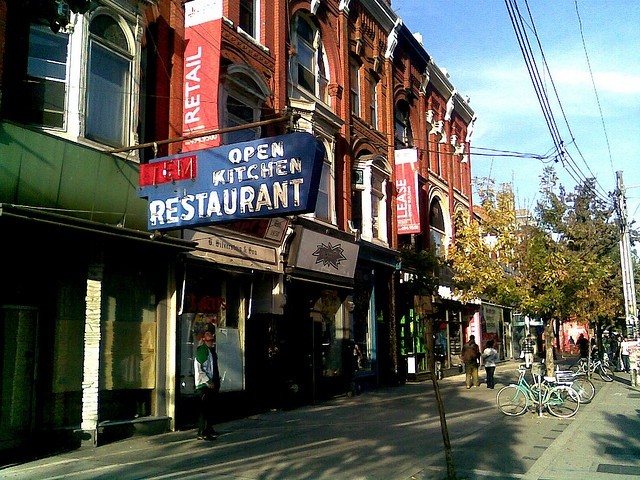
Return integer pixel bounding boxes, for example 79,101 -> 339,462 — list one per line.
198,435 -> 204,439
202,431 -> 219,438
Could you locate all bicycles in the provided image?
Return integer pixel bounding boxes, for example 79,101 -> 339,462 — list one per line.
529,360 -> 595,404
575,360 -> 614,382
497,368 -> 580,418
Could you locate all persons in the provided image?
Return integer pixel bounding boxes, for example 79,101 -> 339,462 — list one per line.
619,338 -> 629,372
576,333 -> 618,362
627,348 -> 640,387
482,341 -> 497,389
520,335 -> 536,369
568,336 -> 574,356
194,330 -> 216,389
460,335 -> 481,388
